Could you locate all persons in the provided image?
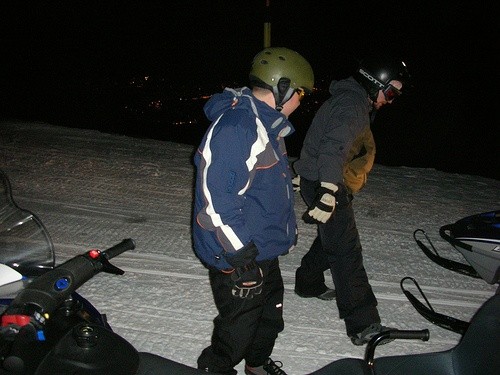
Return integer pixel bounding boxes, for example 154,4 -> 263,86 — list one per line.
193,48 -> 314,375
292,52 -> 412,346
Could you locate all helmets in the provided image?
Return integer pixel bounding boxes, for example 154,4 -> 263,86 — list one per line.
359,49 -> 410,91
249,47 -> 314,92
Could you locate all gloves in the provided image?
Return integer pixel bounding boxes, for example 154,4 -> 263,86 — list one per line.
291,174 -> 301,192
226,240 -> 265,312
280,223 -> 298,256
302,182 -> 338,225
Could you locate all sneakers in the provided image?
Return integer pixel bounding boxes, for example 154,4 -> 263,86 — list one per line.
245,358 -> 287,375
317,289 -> 337,301
350,322 -> 398,346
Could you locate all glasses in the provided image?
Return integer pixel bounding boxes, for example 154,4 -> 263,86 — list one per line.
296,88 -> 308,103
382,84 -> 402,102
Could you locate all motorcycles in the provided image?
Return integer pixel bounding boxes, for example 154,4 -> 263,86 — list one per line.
0,167 -> 500,375
